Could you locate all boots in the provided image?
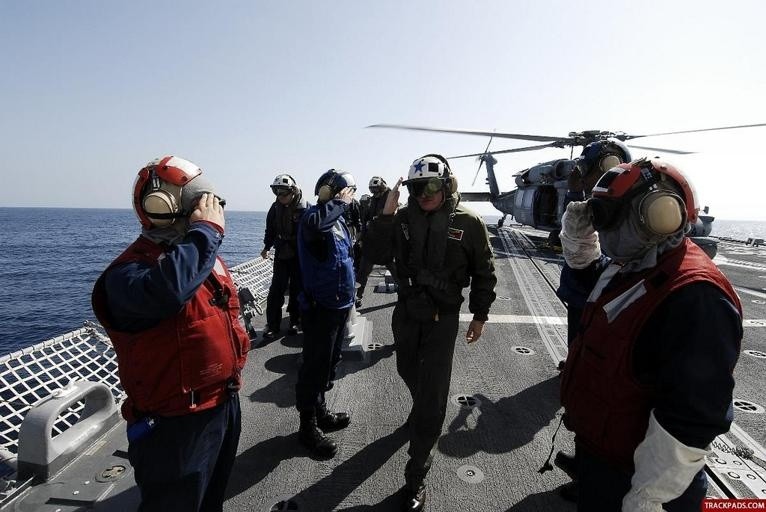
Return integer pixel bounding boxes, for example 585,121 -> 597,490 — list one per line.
298,417 -> 338,455
317,403 -> 350,431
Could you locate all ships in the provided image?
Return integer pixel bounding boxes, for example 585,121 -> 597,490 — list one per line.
1,216 -> 766,510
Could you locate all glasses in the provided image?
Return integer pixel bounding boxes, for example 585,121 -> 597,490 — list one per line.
273,187 -> 294,197
587,175 -> 660,231
410,180 -> 443,197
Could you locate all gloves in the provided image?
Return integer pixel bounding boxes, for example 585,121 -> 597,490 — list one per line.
621,408 -> 712,511
558,197 -> 602,269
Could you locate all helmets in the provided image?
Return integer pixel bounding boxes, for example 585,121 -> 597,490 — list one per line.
132,155 -> 203,234
592,157 -> 697,224
360,195 -> 371,207
369,177 -> 388,193
315,170 -> 357,196
270,174 -> 296,189
581,137 -> 631,163
402,154 -> 454,185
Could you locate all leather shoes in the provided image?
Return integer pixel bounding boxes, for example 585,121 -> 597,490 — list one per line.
263,328 -> 279,339
406,474 -> 426,511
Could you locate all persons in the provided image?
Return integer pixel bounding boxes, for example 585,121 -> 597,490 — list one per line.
366,155 -> 497,510
259,173 -> 315,337
558,157 -> 744,512
294,169 -> 356,459
315,177 -> 399,390
557,136 -> 633,496
91,154 -> 251,512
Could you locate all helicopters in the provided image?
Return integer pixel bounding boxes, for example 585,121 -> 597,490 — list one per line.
363,121 -> 765,253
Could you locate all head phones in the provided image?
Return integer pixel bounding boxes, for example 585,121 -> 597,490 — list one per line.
281,173 -> 300,196
418,154 -> 458,195
637,160 -> 687,238
599,139 -> 624,174
142,156 -> 178,227
318,170 -> 350,202
